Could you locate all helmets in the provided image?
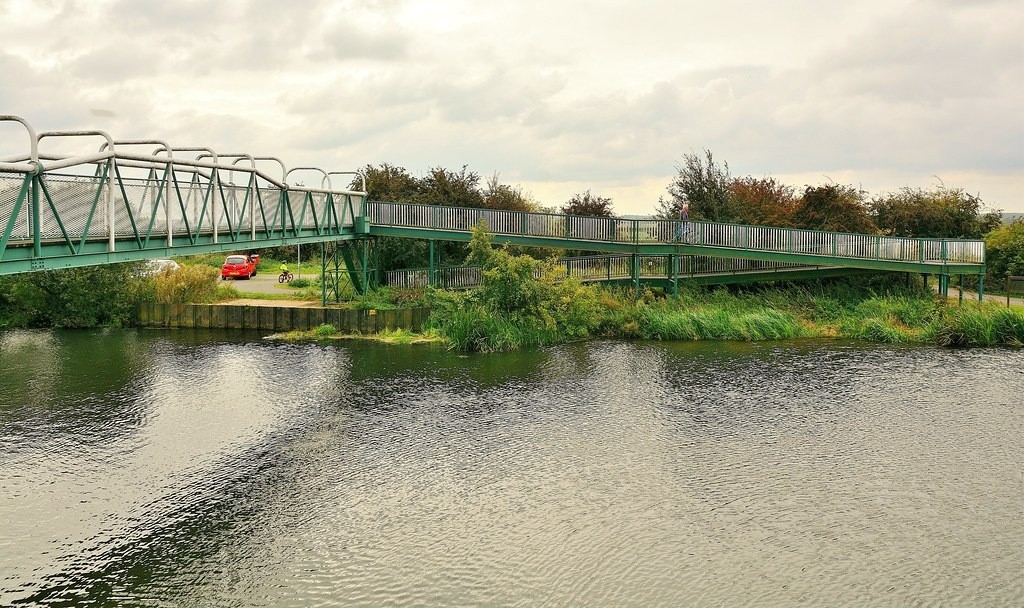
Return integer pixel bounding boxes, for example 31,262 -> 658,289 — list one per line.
282,260 -> 287,264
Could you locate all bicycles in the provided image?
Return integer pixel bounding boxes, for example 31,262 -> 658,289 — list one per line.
279,270 -> 294,283
662,222 -> 701,246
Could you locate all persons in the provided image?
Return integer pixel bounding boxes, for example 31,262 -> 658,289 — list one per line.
680,203 -> 689,219
280,259 -> 289,283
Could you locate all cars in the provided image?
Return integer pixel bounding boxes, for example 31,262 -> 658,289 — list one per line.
221,255 -> 257,280
133,259 -> 178,277
